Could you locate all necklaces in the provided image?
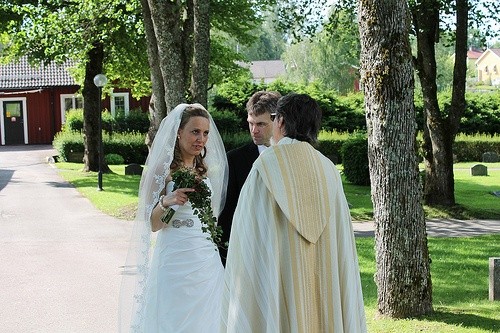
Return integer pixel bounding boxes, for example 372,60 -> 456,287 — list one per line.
180,157 -> 198,171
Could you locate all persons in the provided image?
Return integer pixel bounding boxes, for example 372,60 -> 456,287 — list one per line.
214,89 -> 284,266
130,103 -> 229,333
224,94 -> 368,333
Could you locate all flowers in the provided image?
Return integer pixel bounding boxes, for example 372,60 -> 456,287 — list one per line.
160,164 -> 229,249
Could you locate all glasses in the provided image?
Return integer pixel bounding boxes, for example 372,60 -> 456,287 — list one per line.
270,113 -> 278,121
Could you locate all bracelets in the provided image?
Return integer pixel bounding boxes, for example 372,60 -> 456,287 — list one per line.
158,195 -> 172,211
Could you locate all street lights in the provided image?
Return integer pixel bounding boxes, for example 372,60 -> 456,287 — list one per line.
94,73 -> 108,192
